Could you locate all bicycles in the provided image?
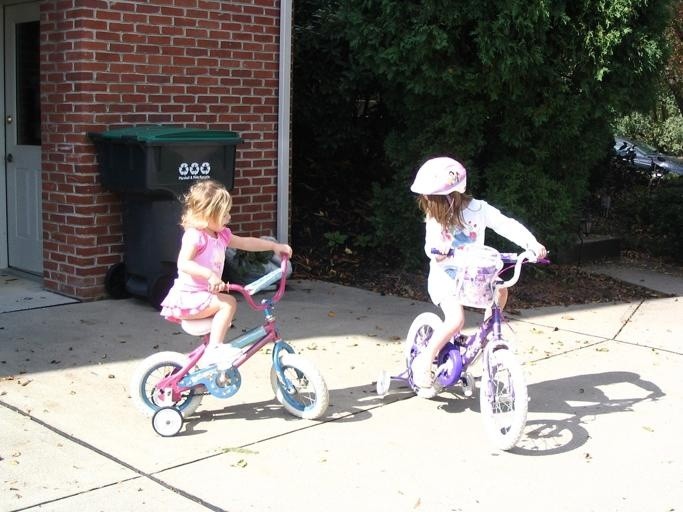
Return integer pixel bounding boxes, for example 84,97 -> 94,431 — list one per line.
129,250 -> 332,439
370,243 -> 552,451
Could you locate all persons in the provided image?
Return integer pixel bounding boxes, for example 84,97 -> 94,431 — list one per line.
409,156 -> 548,389
160,179 -> 293,370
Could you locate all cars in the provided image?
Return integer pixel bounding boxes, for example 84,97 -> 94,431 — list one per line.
612,132 -> 683,187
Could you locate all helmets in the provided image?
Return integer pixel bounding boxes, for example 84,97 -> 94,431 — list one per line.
410,157 -> 467,195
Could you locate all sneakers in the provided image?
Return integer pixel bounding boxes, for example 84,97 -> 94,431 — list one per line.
411,355 -> 433,388
207,343 -> 243,367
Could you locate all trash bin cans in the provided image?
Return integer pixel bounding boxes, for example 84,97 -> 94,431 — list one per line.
86,125 -> 244,312
